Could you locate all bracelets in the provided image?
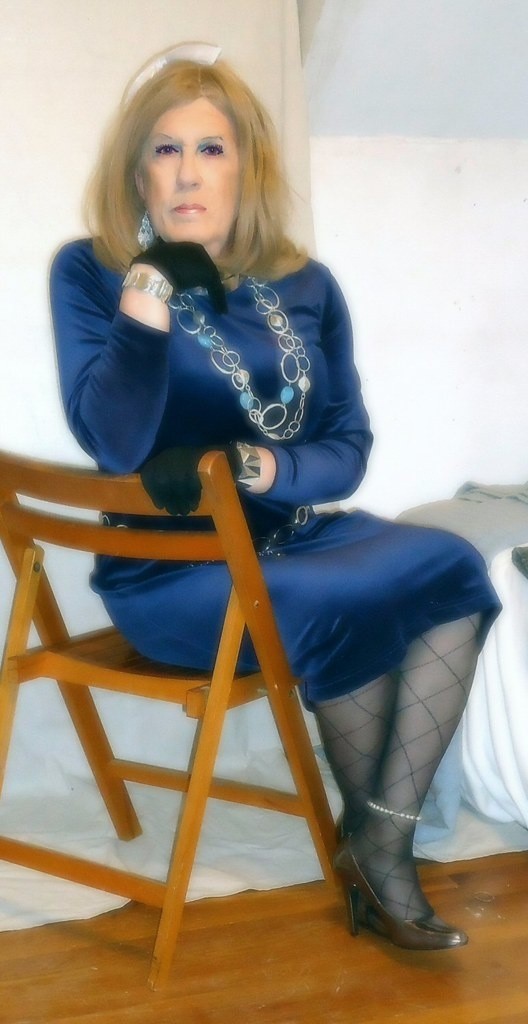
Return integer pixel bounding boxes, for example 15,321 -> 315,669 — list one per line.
230,440 -> 261,490
122,269 -> 176,307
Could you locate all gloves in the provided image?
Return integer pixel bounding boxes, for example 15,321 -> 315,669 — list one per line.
129,237 -> 229,317
140,443 -> 243,516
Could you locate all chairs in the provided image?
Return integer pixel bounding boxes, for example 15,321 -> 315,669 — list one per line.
0,448 -> 349,994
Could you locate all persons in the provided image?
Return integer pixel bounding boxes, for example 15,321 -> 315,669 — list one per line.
50,60 -> 504,950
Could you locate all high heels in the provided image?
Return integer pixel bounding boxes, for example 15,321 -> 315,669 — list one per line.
335,837 -> 468,949
334,811 -> 389,935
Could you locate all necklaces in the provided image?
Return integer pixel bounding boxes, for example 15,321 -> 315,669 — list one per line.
139,212 -> 312,441
